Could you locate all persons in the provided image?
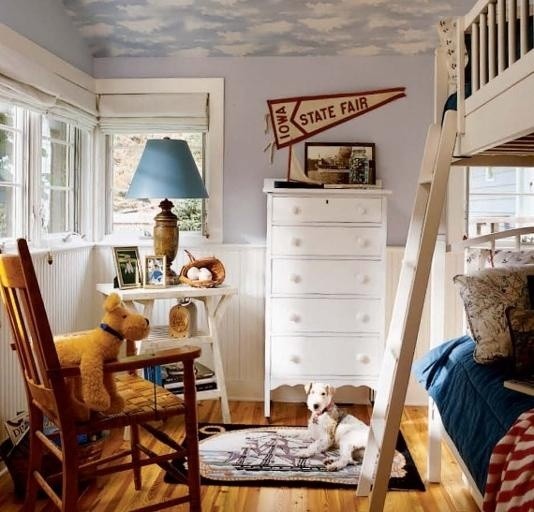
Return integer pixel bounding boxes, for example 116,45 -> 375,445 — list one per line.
318,154 -> 322,166
150,264 -> 162,282
125,256 -> 135,283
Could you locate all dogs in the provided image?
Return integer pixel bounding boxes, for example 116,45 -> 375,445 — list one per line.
295,381 -> 370,471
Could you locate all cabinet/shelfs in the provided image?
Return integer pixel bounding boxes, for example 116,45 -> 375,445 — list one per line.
262,178 -> 389,418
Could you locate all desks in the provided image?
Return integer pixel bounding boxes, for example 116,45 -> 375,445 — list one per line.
96,283 -> 238,441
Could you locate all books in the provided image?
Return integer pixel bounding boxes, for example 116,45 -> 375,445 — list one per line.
144,362 -> 218,395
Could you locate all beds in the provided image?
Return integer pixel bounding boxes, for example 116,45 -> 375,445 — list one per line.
427,0 -> 534,512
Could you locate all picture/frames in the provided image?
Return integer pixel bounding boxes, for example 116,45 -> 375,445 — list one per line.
305,142 -> 375,185
110,245 -> 143,290
143,255 -> 167,289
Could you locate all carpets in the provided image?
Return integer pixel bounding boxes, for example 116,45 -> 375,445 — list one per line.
164,423 -> 426,493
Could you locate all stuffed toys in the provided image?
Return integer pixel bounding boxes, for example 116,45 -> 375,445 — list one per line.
53,292 -> 151,416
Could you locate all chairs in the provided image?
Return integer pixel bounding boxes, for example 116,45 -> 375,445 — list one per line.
0,236 -> 201,512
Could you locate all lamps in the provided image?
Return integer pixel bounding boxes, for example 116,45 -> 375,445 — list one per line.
124,136 -> 209,285
